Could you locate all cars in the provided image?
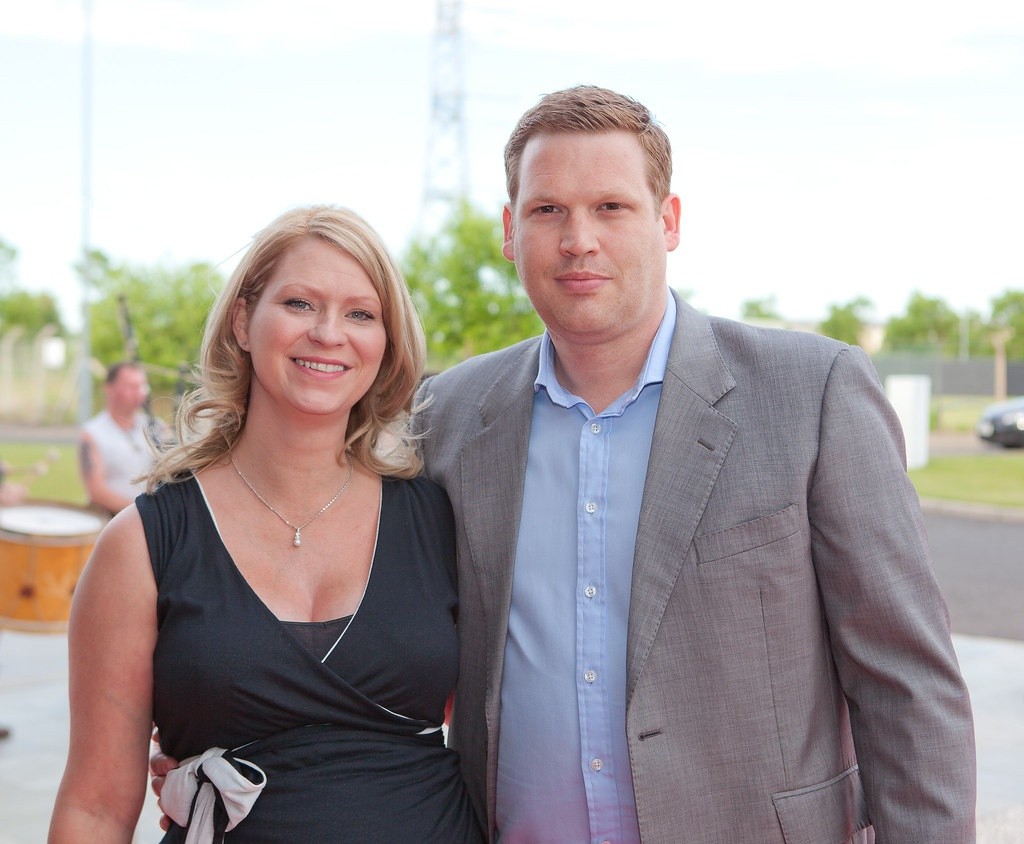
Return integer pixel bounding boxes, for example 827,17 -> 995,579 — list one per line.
978,395 -> 1024,449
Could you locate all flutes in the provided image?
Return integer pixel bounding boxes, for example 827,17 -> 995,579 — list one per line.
115,292 -> 193,457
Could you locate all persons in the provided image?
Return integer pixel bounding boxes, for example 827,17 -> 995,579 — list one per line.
77,363 -> 174,515
150,82 -> 976,844
49,204 -> 486,844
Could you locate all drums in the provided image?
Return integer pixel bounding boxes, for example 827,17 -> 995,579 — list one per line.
1,497 -> 113,635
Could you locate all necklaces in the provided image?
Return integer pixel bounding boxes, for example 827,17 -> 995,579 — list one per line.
227,450 -> 354,547
